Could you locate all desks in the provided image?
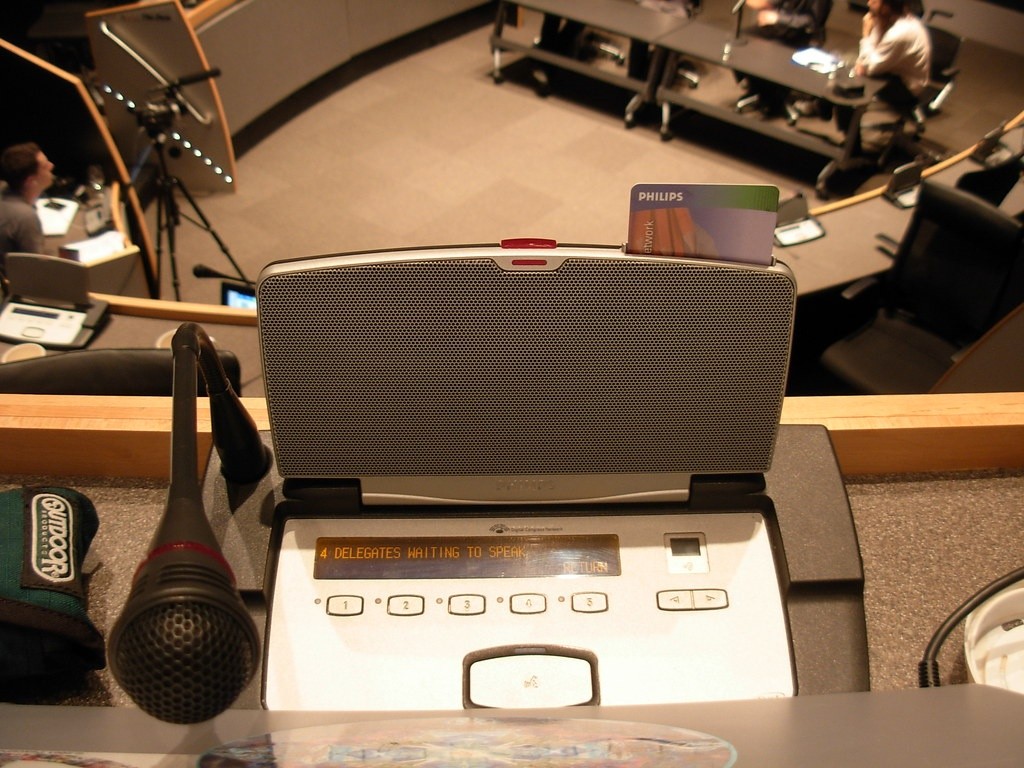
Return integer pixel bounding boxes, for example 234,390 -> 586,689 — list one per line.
773,112 -> 1024,296
655,23 -> 887,200
489,0 -> 689,129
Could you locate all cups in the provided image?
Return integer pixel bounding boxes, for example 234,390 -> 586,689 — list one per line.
2,344 -> 47,364
156,329 -> 215,350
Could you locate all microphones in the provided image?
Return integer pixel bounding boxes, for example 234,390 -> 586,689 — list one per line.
108,322 -> 267,725
174,67 -> 222,86
193,264 -> 258,284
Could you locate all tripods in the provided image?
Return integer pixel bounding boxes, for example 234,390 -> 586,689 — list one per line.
151,134 -> 247,301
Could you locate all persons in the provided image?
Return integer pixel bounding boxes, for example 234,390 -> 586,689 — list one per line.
727,0 -> 830,117
0,142 -> 57,297
842,0 -> 929,157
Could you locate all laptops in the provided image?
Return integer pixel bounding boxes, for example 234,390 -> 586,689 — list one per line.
220,282 -> 257,311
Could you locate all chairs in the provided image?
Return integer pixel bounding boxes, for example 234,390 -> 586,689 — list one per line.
819,182 -> 1024,395
876,23 -> 964,173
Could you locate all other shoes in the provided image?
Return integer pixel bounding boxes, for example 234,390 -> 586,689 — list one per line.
794,101 -> 832,121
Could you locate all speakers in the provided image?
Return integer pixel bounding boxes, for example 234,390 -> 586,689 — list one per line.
257,240 -> 796,509
